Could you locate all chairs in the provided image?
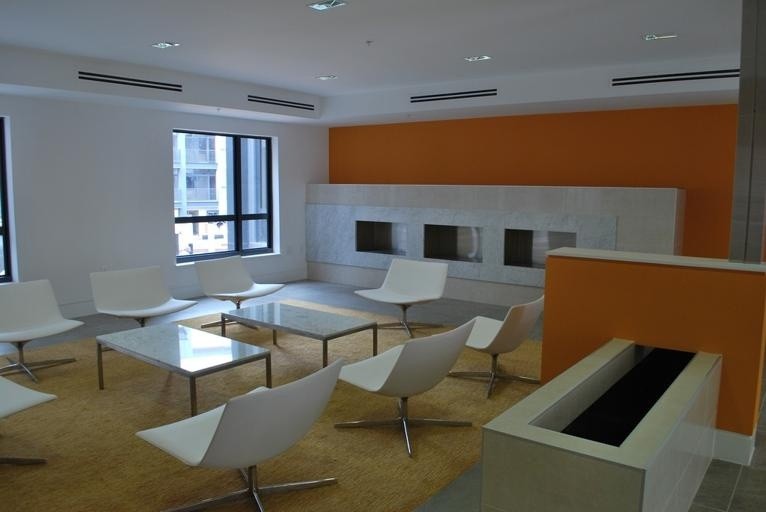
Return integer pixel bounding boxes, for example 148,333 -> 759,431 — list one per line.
89,266 -> 198,328
354,258 -> 449,339
0,375 -> 59,465
447,296 -> 550,400
193,254 -> 286,333
333,317 -> 478,458
135,355 -> 346,512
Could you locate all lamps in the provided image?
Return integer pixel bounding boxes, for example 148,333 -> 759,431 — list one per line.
463,56 -> 491,63
152,41 -> 178,51
306,1 -> 345,11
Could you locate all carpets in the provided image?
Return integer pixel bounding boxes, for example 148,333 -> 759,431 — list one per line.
0,302 -> 543,511
